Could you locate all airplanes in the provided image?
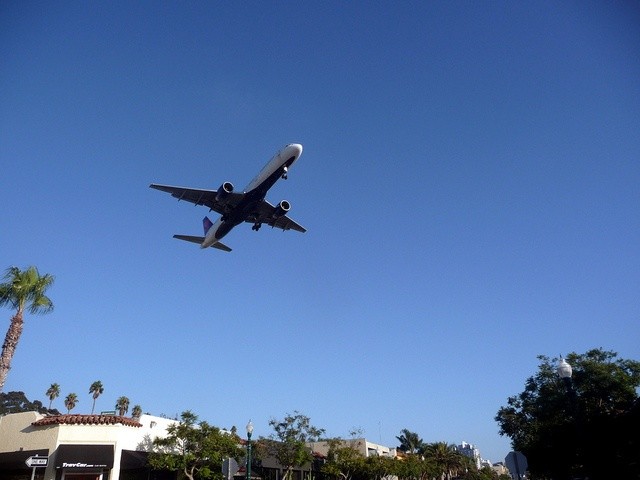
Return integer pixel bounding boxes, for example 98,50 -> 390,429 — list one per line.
149,143 -> 307,253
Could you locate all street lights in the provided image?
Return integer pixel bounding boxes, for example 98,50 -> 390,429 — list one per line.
556,357 -> 599,480
246,419 -> 254,480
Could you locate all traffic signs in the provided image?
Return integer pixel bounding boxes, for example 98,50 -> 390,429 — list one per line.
24,456 -> 49,468
